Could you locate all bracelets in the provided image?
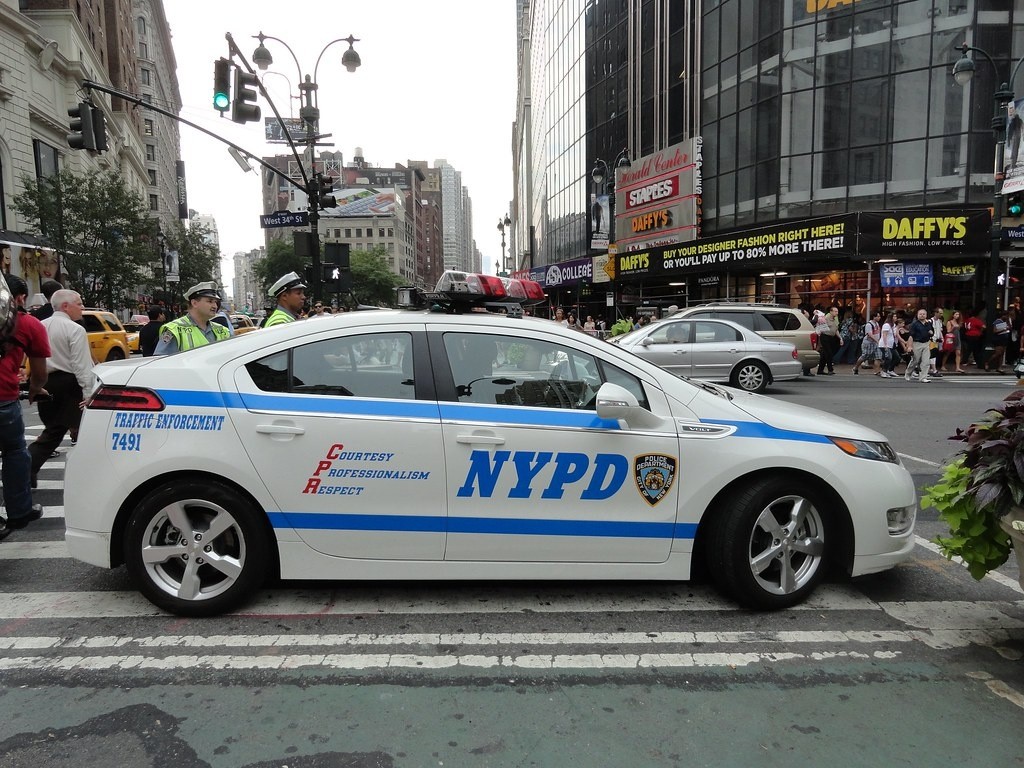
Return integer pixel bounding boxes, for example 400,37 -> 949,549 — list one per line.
20,365 -> 26,369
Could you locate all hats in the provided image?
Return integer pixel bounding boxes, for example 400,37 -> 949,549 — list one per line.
183,281 -> 221,301
268,271 -> 307,297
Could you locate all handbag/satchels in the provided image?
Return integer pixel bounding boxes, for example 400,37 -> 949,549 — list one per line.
943,333 -> 955,350
813,311 -> 830,335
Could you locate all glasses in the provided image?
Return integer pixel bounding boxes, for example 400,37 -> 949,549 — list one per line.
315,306 -> 322,308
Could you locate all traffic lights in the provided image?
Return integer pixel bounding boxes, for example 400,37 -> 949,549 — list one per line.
318,173 -> 336,209
66,102 -> 97,151
324,265 -> 340,282
232,68 -> 261,125
213,59 -> 231,111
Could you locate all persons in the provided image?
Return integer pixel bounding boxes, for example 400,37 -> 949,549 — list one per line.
140,305 -> 188,357
593,202 -> 603,234
848,295 -> 866,315
153,281 -> 234,357
1008,114 -> 1023,168
878,293 -> 896,312
524,307 -> 607,365
830,294 -> 842,307
0,274 -> 51,540
17,281 -> 99,488
801,305 -> 1024,383
996,297 -> 1021,310
167,253 -> 174,272
0,243 -> 59,308
258,271 -> 406,368
626,315 -> 657,332
668,305 -> 678,314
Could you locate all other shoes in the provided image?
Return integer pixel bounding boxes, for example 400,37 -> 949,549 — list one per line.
880,370 -> 891,378
817,371 -> 828,375
50,451 -> 60,457
71,438 -> 77,445
956,368 -> 965,372
996,368 -> 1004,372
941,367 -> 947,371
911,372 -> 919,379
828,372 -> 834,375
919,378 -> 932,383
905,369 -> 910,381
887,370 -> 899,376
7,504 -> 43,529
933,370 -> 943,377
803,372 -> 815,376
31,471 -> 37,488
852,367 -> 858,376
984,362 -> 989,372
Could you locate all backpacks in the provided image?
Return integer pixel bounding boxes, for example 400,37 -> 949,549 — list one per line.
596,321 -> 604,333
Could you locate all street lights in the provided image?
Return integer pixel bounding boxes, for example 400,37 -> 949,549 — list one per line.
251,30 -> 362,314
497,212 -> 511,272
591,147 -> 632,332
950,41 -> 1024,373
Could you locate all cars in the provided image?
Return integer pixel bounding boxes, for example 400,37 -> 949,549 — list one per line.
609,317 -> 804,394
649,300 -> 821,371
60,266 -> 920,617
75,306 -> 150,366
212,311 -> 266,336
